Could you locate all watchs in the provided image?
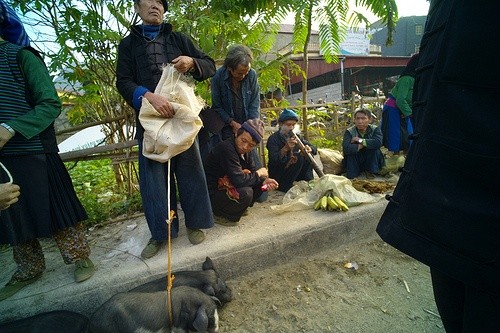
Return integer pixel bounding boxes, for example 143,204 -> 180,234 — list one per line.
359,139 -> 363,143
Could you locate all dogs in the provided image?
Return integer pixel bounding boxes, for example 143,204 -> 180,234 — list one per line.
0,255 -> 235,332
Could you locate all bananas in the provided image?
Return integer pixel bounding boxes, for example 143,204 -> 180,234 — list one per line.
313,189 -> 349,212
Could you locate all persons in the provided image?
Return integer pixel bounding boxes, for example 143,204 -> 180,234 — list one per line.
0,183 -> 20,211
342,108 -> 385,179
380,53 -> 420,156
0,0 -> 95,301
211,44 -> 268,203
201,118 -> 279,226
116,0 -> 216,258
266,108 -> 317,193
376,0 -> 500,333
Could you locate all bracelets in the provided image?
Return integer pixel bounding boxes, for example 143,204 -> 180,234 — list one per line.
0,123 -> 15,136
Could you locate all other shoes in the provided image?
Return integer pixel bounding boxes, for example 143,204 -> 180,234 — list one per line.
215,212 -> 242,221
186,226 -> 205,244
0,273 -> 40,301
140,239 -> 163,259
75,258 -> 94,282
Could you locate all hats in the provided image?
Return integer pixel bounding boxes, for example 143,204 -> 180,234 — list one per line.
243,119 -> 265,143
278,109 -> 300,120
133,0 -> 168,12
